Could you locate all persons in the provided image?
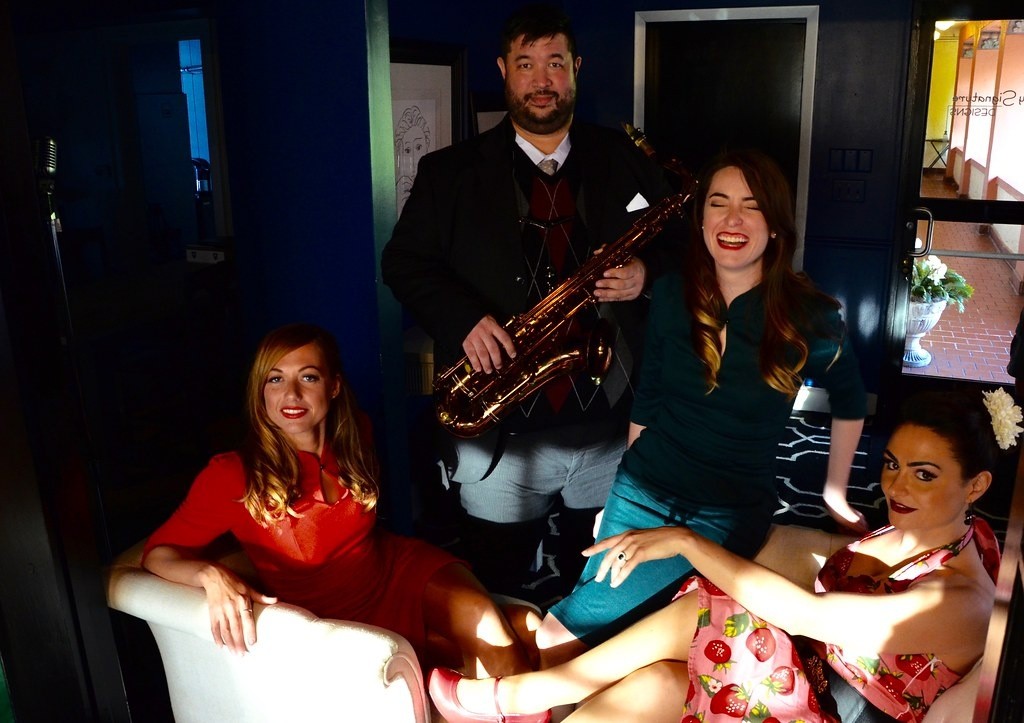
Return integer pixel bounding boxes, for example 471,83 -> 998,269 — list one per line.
381,15 -> 677,609
142,322 -> 543,723
535,148 -> 868,723
424,390 -> 995,723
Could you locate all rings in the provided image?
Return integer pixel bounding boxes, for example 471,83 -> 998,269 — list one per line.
616,552 -> 628,563
240,609 -> 253,612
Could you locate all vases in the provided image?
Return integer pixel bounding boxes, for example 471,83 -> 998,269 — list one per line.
902,293 -> 951,368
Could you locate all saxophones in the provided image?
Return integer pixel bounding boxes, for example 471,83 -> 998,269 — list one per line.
430,117 -> 703,443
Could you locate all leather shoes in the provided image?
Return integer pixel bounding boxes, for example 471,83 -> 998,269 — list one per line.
427,666 -> 552,723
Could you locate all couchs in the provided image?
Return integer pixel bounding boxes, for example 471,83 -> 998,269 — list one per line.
755,522 -> 986,723
102,534 -> 543,723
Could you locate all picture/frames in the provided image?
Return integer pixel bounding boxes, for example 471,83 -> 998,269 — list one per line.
389,36 -> 464,220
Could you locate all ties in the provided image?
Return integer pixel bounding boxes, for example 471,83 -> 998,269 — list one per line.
538,159 -> 558,176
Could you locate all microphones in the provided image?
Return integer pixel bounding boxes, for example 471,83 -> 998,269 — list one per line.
25,134 -> 62,234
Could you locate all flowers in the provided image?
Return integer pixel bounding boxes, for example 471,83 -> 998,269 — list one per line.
910,238 -> 975,314
981,387 -> 1024,450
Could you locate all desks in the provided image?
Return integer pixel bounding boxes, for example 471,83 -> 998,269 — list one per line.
925,139 -> 951,173
